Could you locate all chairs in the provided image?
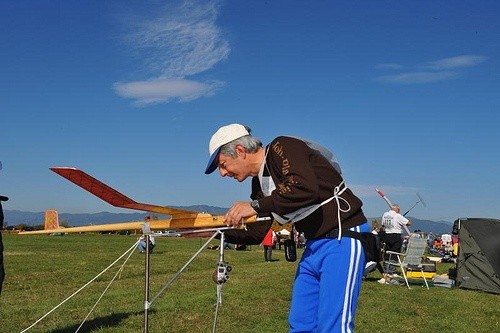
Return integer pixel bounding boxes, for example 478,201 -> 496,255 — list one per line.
384,237 -> 435,290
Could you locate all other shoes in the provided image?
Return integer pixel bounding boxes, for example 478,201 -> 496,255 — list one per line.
377,278 -> 400,284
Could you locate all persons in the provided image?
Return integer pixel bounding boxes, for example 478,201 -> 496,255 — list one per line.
371,226 -> 456,259
136,230 -> 155,255
380,205 -> 411,275
261,227 -> 276,262
204,123 -> 370,333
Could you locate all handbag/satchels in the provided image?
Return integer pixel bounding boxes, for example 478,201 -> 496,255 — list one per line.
326,227 -> 383,262
284,238 -> 297,262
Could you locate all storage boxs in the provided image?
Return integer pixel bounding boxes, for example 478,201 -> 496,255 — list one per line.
433,277 -> 455,288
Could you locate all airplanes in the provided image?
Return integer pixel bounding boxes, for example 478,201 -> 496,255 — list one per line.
374,187 -> 427,237
0,165 -> 256,236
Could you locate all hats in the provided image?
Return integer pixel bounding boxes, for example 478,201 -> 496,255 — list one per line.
204,123 -> 251,174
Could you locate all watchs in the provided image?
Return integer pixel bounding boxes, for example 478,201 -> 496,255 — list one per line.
250,200 -> 260,213
